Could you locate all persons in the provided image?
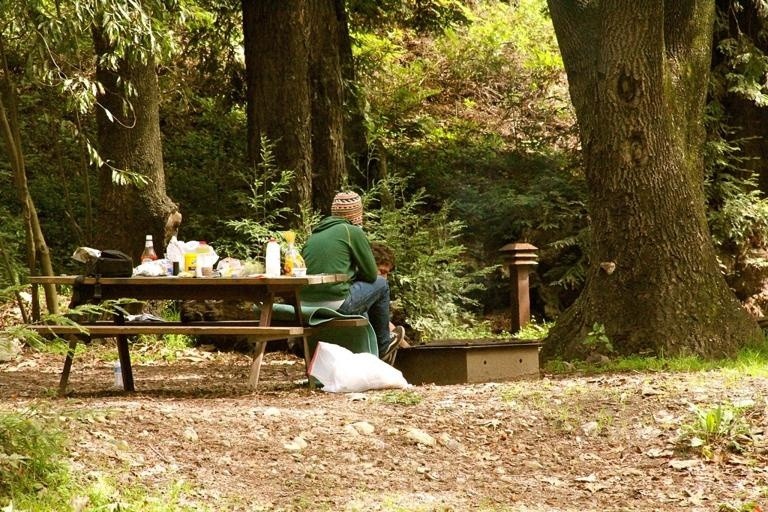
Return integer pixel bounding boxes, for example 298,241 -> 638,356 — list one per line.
291,190 -> 406,366
368,240 -> 410,347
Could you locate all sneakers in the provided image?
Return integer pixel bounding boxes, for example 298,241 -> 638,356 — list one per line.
377,325 -> 405,367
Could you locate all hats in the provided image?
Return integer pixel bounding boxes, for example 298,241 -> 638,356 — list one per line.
329,191 -> 364,227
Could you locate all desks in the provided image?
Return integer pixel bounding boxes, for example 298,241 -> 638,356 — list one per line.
26,273 -> 348,396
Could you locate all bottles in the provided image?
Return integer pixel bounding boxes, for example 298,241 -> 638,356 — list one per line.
195,240 -> 213,278
266,238 -> 281,278
140,235 -> 158,265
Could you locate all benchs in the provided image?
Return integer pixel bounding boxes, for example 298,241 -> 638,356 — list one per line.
24,316 -> 371,395
